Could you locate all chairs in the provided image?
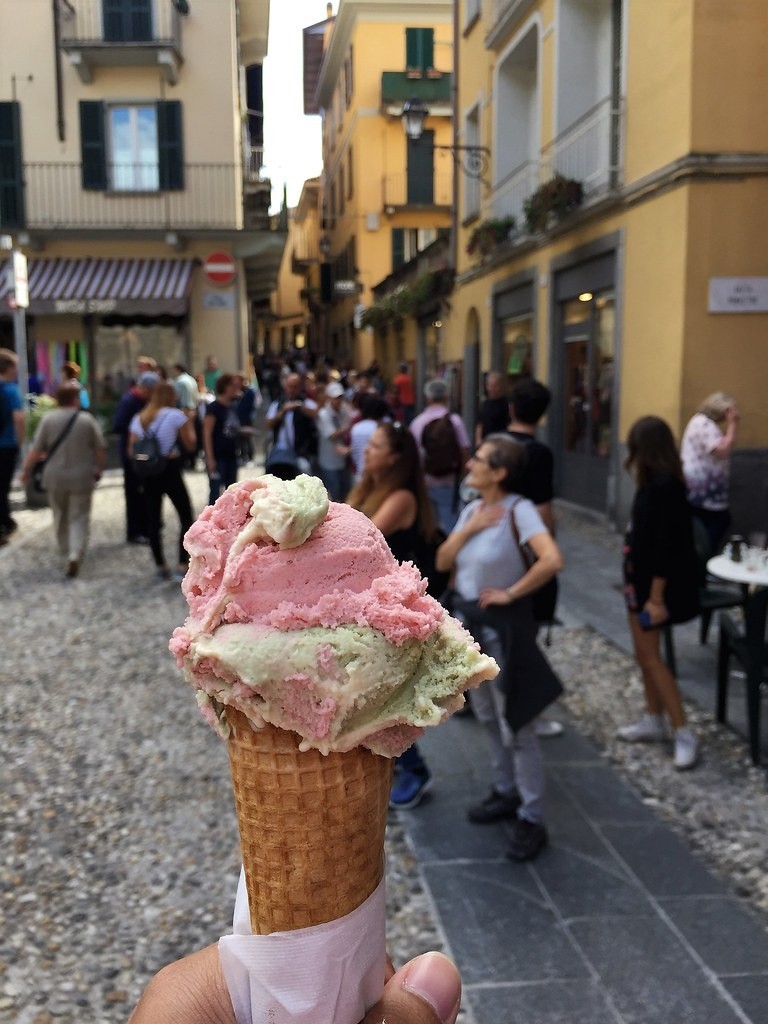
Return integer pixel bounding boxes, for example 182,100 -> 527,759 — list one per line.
663,531 -> 768,769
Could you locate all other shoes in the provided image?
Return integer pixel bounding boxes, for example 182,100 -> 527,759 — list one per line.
157,565 -> 170,577
503,818 -> 547,863
65,553 -> 80,577
0,519 -> 18,545
467,788 -> 521,825
177,563 -> 189,574
533,718 -> 564,737
617,715 -> 671,744
670,727 -> 700,770
126,534 -> 148,544
388,761 -> 435,809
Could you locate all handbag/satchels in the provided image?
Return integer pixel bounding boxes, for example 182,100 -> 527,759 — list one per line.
510,496 -> 562,627
31,459 -> 47,494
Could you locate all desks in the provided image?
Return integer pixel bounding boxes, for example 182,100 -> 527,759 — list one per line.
706,553 -> 768,615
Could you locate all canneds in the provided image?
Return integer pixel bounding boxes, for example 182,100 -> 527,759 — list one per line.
730,534 -> 744,561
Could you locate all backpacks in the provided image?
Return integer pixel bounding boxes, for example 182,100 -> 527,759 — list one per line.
420,410 -> 463,479
0,381 -> 16,435
132,409 -> 179,482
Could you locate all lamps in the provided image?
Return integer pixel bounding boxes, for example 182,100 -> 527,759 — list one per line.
319,235 -> 350,263
401,97 -> 492,187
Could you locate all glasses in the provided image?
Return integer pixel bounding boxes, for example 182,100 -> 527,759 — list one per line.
471,453 -> 491,468
382,415 -> 407,439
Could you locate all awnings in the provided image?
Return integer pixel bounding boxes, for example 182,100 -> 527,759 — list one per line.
0,257 -> 195,318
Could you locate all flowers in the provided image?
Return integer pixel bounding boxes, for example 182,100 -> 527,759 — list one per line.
522,171 -> 584,232
358,265 -> 456,330
464,214 -> 517,257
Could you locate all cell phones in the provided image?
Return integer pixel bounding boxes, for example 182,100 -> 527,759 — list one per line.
640,612 -> 650,630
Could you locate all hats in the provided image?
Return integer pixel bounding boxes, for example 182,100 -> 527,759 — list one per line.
323,381 -> 345,399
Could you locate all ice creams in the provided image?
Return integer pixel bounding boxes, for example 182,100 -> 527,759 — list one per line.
165,474 -> 500,933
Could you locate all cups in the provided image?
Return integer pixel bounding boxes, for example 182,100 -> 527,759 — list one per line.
743,551 -> 761,571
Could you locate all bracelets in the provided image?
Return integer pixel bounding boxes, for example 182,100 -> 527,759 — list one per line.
649,597 -> 664,605
504,587 -> 518,601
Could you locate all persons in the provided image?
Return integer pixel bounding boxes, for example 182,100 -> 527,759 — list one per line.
343,416 -> 445,600
125,942 -> 463,1024
253,347 -> 418,507
409,379 -> 474,532
0,347 -> 24,544
569,361 -> 609,458
611,415 -> 709,767
16,363 -> 103,577
477,371 -> 513,449
433,434 -> 564,860
103,355 -> 259,581
676,390 -> 741,566
